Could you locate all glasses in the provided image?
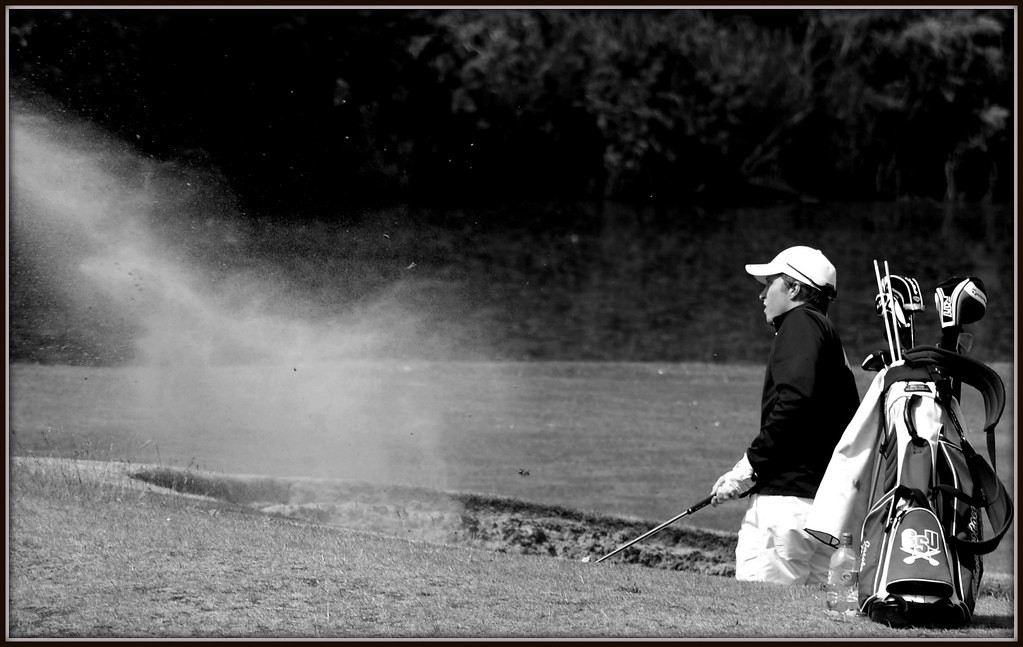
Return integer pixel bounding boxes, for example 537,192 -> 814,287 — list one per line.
787,262 -> 841,305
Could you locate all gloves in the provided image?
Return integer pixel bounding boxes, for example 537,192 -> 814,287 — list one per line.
711,469 -> 738,507
730,454 -> 756,495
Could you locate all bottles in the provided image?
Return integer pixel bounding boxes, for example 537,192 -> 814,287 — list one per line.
825,531 -> 860,620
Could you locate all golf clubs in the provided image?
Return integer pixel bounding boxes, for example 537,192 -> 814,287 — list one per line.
594,472 -> 759,564
860,258 -> 985,382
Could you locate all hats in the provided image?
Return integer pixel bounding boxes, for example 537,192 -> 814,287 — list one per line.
744,245 -> 836,302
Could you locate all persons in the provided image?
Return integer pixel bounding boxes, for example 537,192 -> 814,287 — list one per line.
710,244 -> 862,589
